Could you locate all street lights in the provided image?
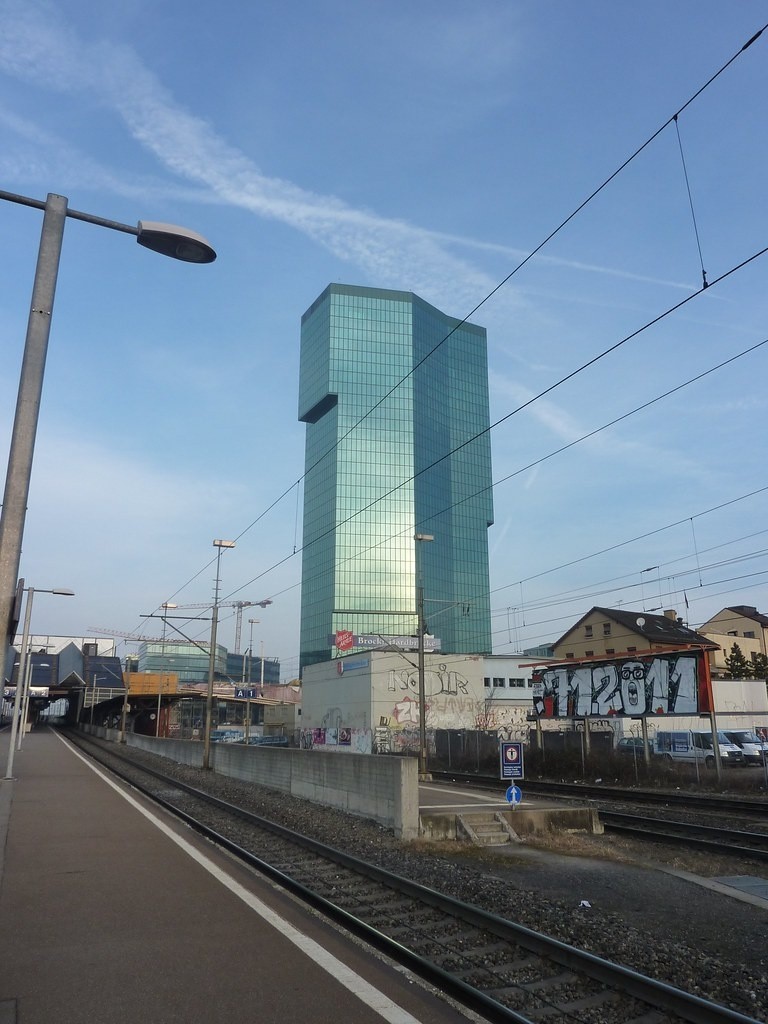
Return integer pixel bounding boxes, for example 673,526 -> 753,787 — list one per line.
90,678 -> 107,734
412,533 -> 434,776
0,180 -> 217,692
202,537 -> 237,772
10,642 -> 56,751
246,619 -> 261,690
2,585 -> 76,780
156,603 -> 178,736
23,663 -> 51,738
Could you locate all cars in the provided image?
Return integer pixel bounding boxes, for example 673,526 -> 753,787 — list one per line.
616,736 -> 654,758
212,729 -> 288,747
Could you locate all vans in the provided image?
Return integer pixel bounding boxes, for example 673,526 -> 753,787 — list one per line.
724,729 -> 768,767
652,730 -> 747,770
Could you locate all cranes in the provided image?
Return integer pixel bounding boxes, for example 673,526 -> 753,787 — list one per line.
159,599 -> 273,656
86,626 -> 207,645
